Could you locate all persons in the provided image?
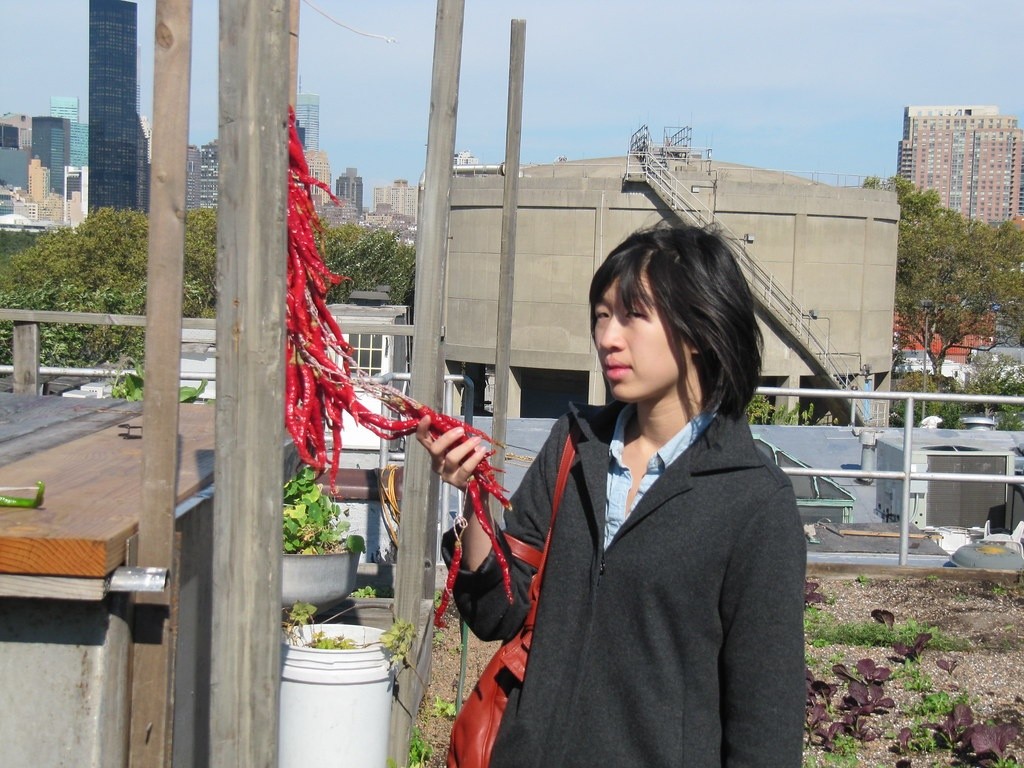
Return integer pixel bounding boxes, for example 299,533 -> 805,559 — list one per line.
416,227 -> 807,768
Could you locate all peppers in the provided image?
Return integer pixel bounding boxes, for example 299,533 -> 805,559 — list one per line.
0,481 -> 45,507
285,106 -> 515,627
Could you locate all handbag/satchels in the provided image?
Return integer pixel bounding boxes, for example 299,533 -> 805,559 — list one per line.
446,602 -> 538,768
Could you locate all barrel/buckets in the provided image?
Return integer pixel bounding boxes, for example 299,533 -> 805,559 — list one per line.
280,624 -> 394,768
